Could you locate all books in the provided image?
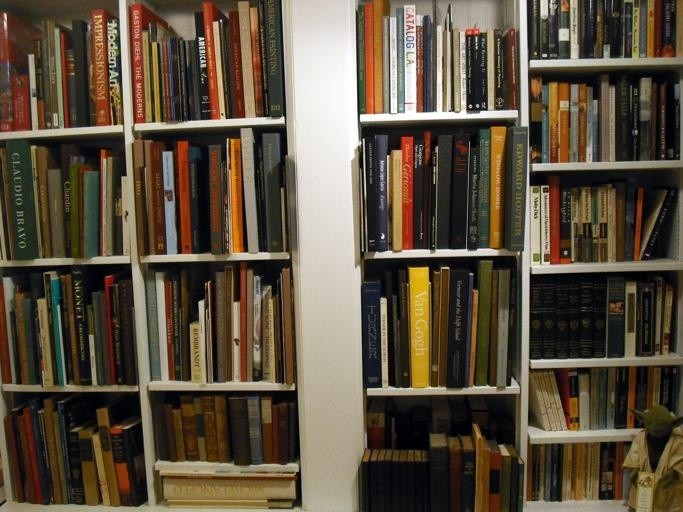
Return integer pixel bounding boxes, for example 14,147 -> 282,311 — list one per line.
2,1 -> 285,129
528,171 -> 678,360
4,391 -> 300,509
530,364 -> 681,501
359,257 -> 525,511
0,135 -> 292,258
0,260 -> 296,388
356,1 -> 527,253
527,0 -> 681,164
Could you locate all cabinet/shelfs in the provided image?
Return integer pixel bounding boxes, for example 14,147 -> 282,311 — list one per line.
356,0 -> 683,512
0,0 -> 303,512
